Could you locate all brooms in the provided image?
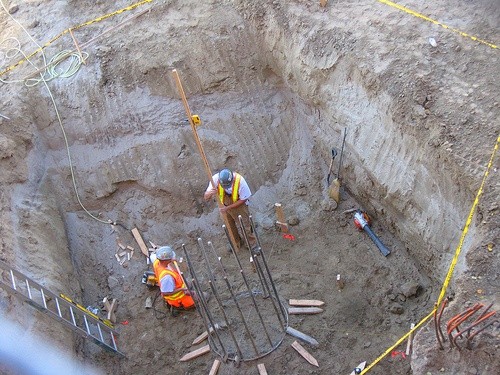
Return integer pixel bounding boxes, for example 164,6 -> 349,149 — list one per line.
328,127 -> 348,202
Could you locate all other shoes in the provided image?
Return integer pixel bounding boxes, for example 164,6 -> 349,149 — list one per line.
167,301 -> 185,317
248,243 -> 261,256
226,241 -> 233,253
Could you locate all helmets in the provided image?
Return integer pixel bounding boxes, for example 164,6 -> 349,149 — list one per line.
156,246 -> 176,261
218,167 -> 234,190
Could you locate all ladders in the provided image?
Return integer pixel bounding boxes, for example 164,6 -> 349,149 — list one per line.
0,260 -> 130,362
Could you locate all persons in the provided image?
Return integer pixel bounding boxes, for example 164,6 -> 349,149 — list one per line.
153,246 -> 197,315
204,169 -> 256,252
